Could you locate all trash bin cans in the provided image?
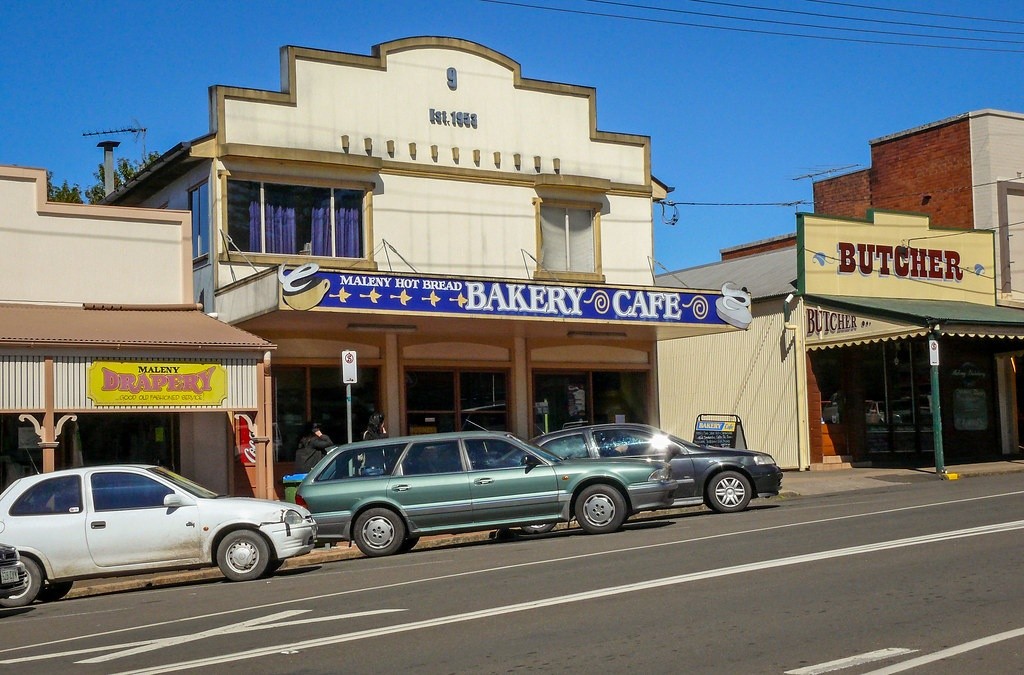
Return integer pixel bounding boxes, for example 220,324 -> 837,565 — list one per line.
283,472 -> 309,505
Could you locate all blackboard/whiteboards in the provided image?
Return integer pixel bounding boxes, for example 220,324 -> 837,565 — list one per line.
691,413 -> 748,451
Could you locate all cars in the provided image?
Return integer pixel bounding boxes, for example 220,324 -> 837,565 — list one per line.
1,543 -> 28,601
0,463 -> 317,610
295,419 -> 679,558
822,395 -> 938,431
496,423 -> 785,536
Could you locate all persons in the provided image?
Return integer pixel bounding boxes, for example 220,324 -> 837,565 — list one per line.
295,421 -> 335,475
362,411 -> 388,469
